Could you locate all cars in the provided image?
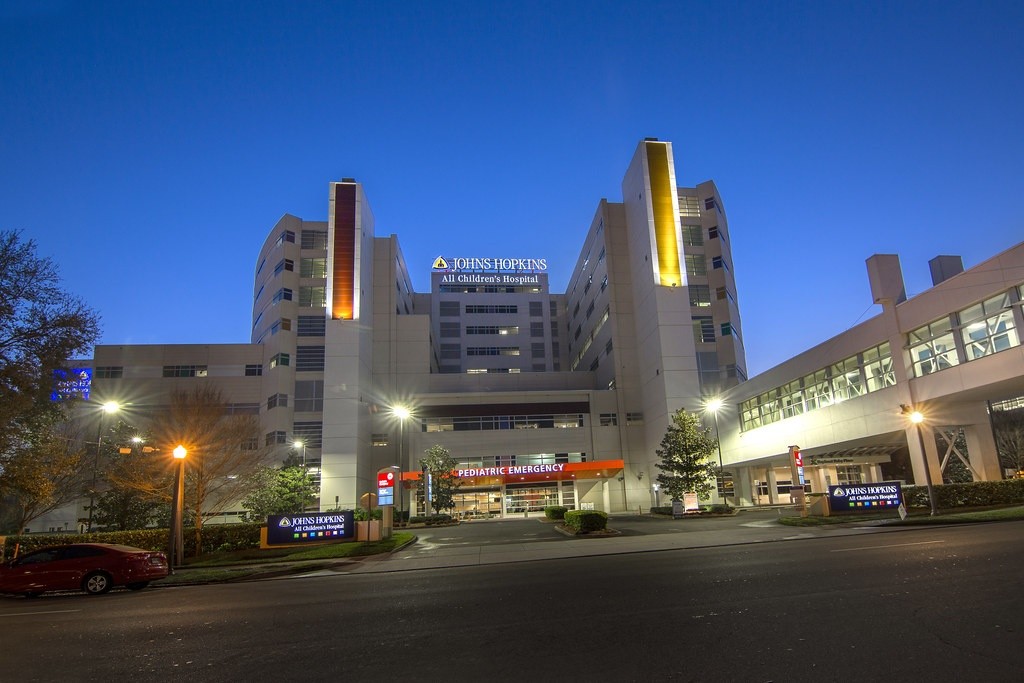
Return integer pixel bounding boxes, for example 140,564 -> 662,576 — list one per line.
0,540 -> 168,598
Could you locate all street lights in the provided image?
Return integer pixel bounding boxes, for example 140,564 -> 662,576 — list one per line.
294,441 -> 306,513
910,411 -> 941,517
166,444 -> 187,575
86,401 -> 120,535
393,405 -> 410,527
706,398 -> 727,504
